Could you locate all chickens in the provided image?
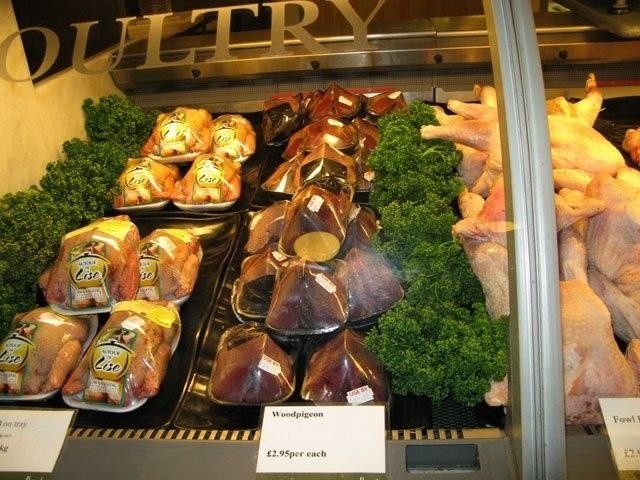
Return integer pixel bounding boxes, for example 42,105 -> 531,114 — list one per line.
111,106 -> 257,208
419,72 -> 640,427
0,214 -> 200,407
